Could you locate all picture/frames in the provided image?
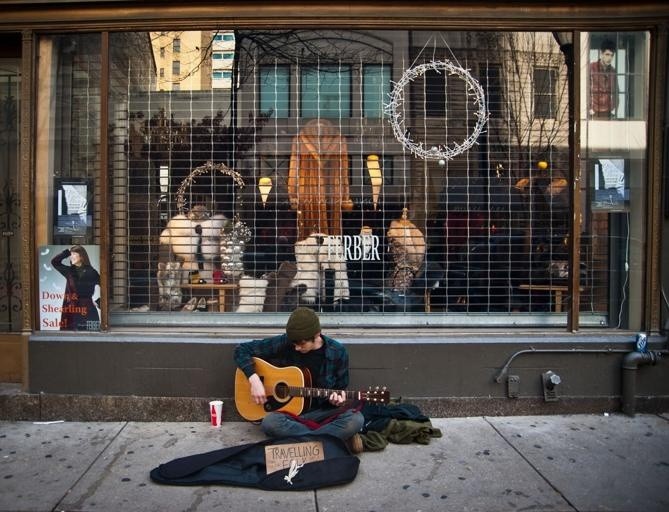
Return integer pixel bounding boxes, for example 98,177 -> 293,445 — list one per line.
54,178 -> 94,245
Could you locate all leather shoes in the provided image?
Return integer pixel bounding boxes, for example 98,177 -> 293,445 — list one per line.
183,297 -> 206,311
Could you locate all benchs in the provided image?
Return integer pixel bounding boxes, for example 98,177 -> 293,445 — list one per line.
172,283 -> 237,313
518,284 -> 584,312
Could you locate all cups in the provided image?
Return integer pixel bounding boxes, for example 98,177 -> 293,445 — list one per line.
208,399 -> 223,430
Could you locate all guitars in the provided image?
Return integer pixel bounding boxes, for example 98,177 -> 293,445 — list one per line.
233,355 -> 389,423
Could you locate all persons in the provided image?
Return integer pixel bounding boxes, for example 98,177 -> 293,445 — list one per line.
513,160 -> 567,262
588,39 -> 619,120
51,245 -> 101,332
355,152 -> 398,234
243,175 -> 289,255
233,308 -> 365,453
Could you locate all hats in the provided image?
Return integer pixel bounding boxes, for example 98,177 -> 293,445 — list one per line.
287,308 -> 320,340
